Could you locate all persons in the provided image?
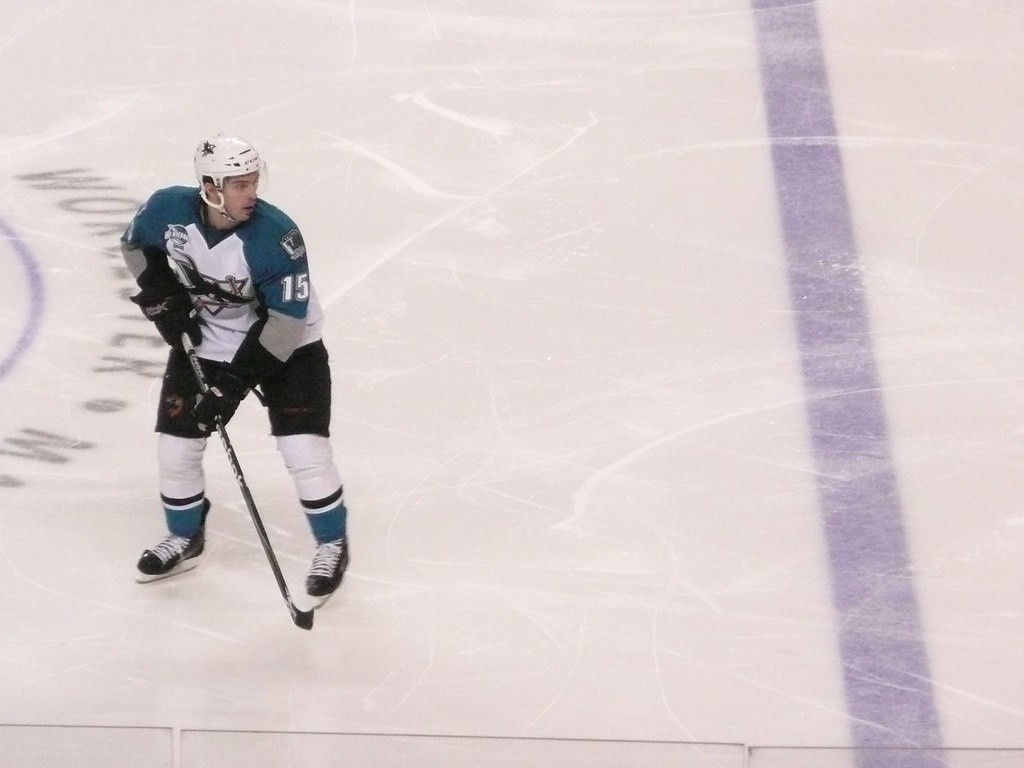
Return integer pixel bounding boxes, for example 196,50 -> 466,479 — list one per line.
119,134 -> 350,607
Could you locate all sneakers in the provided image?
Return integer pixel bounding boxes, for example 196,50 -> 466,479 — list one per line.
305,536 -> 349,608
134,497 -> 209,583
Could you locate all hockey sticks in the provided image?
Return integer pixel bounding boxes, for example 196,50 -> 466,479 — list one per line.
180,331 -> 312,631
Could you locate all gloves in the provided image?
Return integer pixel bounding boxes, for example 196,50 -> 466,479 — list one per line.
129,270 -> 203,352
190,361 -> 255,433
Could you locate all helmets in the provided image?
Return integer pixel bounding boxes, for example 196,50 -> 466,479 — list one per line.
193,132 -> 260,208
